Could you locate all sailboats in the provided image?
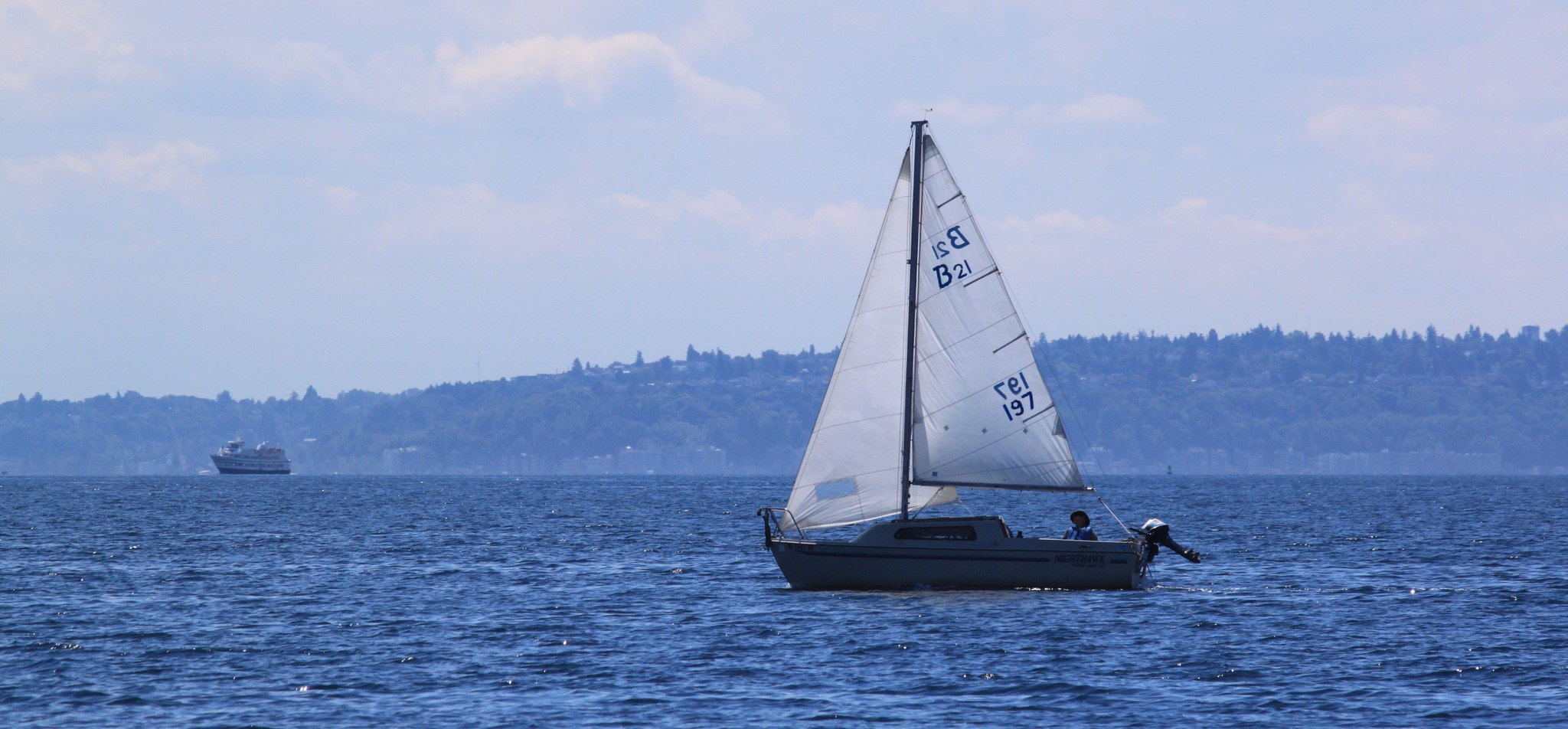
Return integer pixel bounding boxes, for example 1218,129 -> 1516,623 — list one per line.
753,117 -> 1204,593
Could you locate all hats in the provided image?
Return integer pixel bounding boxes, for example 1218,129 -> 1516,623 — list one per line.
1070,510 -> 1090,527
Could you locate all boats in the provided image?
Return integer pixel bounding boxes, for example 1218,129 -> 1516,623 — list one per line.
209,435 -> 291,475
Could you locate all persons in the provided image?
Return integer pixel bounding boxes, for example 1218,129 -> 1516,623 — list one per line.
1062,511 -> 1099,541
